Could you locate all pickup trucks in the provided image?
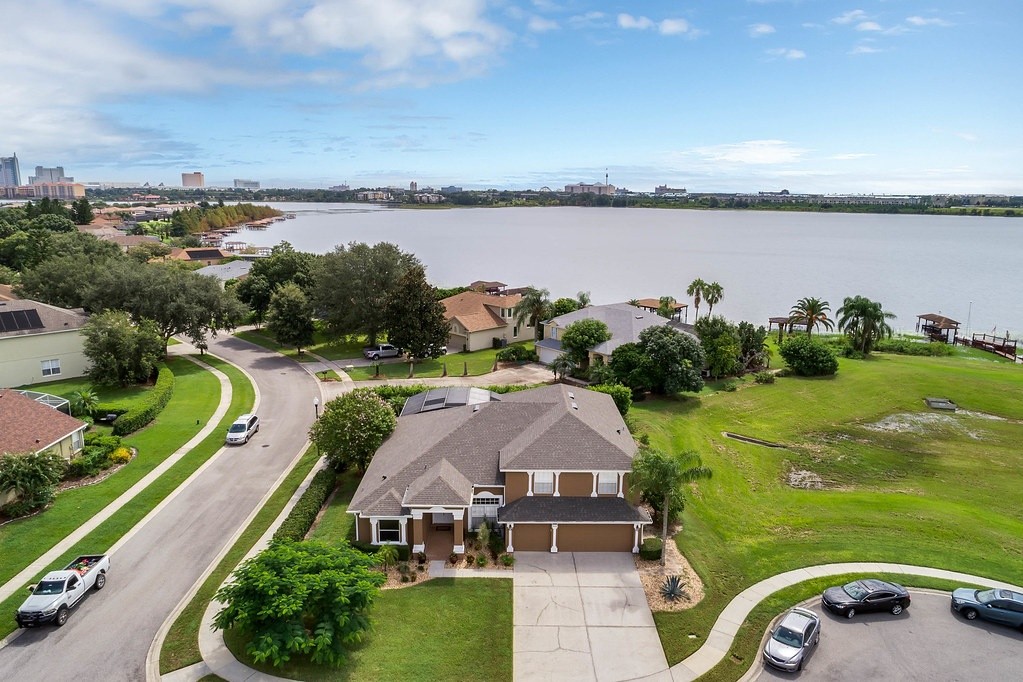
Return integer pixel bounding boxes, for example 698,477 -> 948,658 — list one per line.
13,554 -> 112,630
363,344 -> 405,361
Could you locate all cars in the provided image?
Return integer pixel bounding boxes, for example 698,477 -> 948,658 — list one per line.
420,342 -> 448,358
225,414 -> 260,445
950,587 -> 1023,634
821,578 -> 911,619
761,606 -> 821,673
